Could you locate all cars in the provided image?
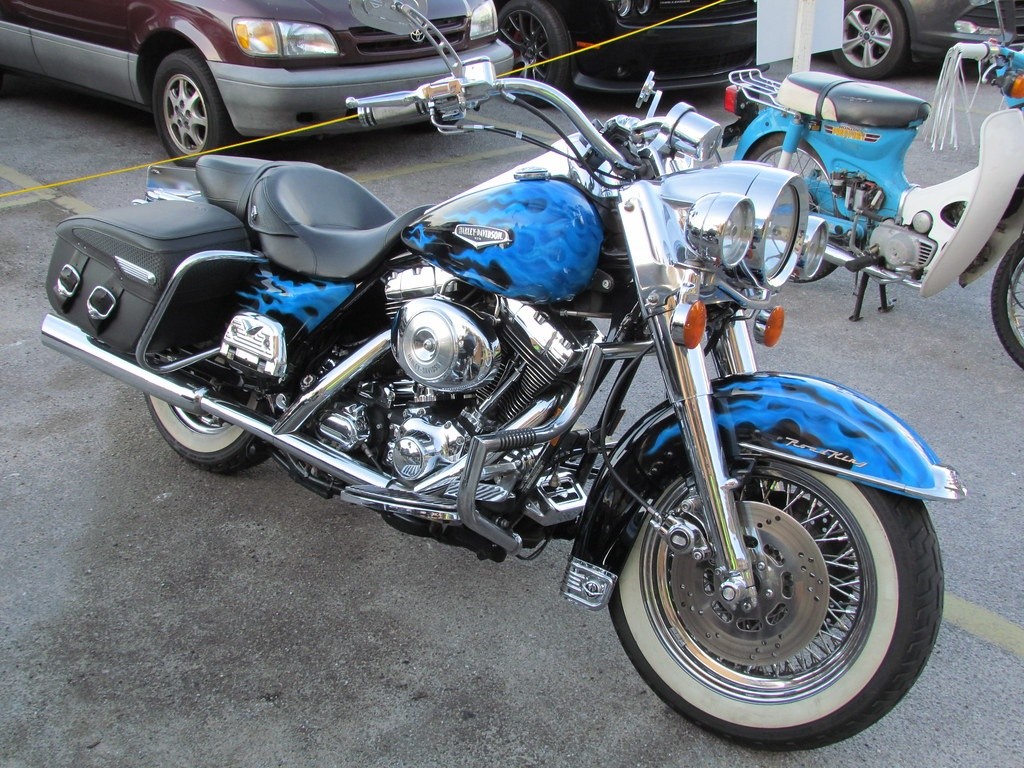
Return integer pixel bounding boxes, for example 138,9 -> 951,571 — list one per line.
494,0 -> 1024,108
0,0 -> 517,167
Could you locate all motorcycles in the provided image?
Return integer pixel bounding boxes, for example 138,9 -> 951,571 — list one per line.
37,0 -> 965,749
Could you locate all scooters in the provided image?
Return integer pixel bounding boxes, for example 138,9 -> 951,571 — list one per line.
723,39 -> 1024,369
